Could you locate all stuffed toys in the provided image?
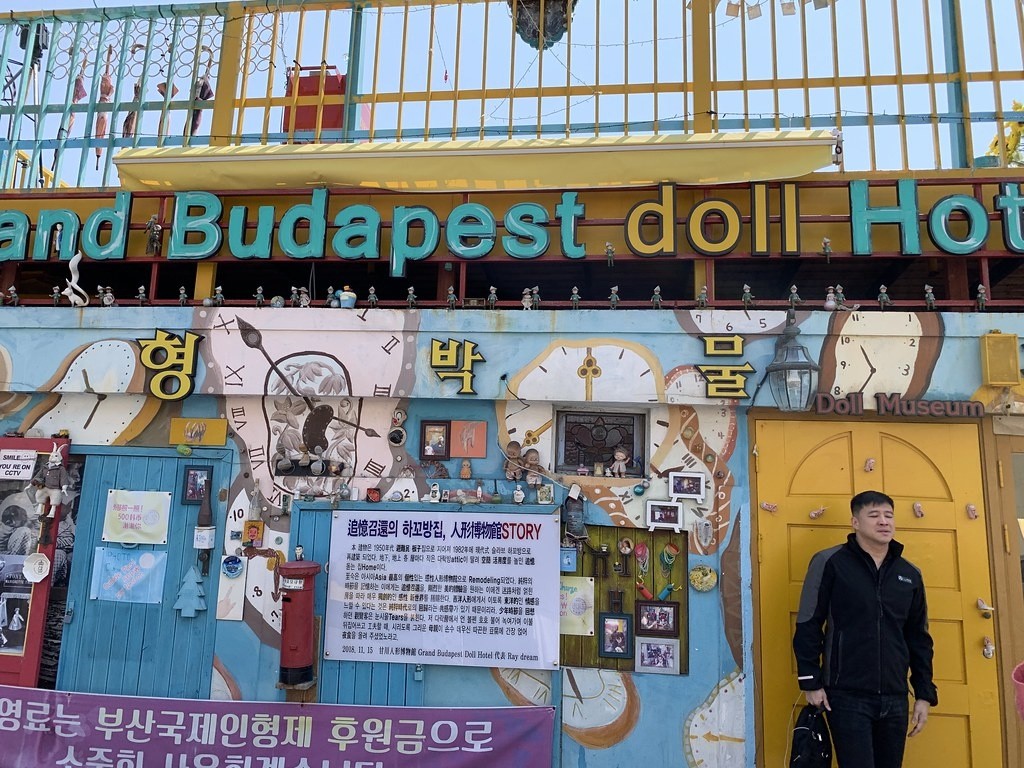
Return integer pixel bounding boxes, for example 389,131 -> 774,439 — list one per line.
24,441 -> 68,517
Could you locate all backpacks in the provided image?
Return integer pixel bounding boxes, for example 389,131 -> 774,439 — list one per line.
789,702 -> 833,768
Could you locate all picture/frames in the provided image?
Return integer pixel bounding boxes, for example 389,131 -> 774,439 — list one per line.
419,420 -> 451,461
635,600 -> 679,637
181,464 -> 213,504
668,472 -> 706,504
635,637 -> 680,675
646,501 -> 682,533
599,611 -> 633,659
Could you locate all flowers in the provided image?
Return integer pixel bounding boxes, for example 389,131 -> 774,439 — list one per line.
986,100 -> 1024,168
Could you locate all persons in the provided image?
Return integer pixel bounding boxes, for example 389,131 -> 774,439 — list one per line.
611,446 -> 630,477
522,238 -> 988,312
1,215 -> 498,310
503,441 -> 545,489
793,491 -> 938,768
460,459 -> 471,480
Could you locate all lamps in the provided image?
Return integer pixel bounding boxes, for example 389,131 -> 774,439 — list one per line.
765,309 -> 821,413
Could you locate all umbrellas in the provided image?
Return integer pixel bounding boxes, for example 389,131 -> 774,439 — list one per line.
53,44 -> 213,170
